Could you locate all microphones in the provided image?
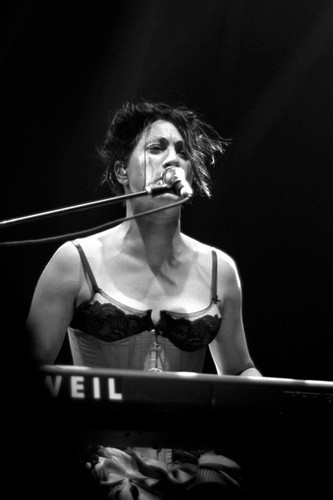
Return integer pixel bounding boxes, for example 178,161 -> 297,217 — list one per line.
163,166 -> 193,197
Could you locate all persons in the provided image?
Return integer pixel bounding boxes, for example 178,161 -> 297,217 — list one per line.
27,99 -> 265,500
